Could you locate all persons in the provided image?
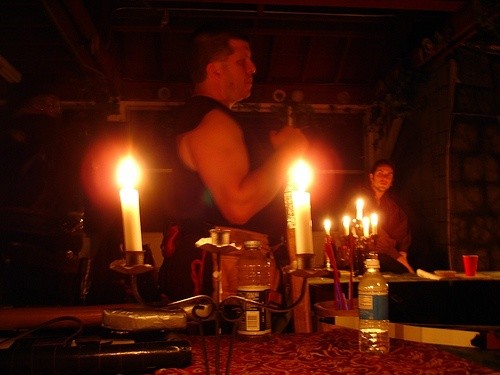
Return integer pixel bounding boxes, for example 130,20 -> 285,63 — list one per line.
155,25 -> 307,304
348,159 -> 415,304
0,68 -> 86,306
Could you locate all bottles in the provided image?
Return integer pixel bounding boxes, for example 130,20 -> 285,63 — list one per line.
237,240 -> 271,340
357,259 -> 389,354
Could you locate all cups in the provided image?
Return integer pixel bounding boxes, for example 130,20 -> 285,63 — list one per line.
463,255 -> 478,277
